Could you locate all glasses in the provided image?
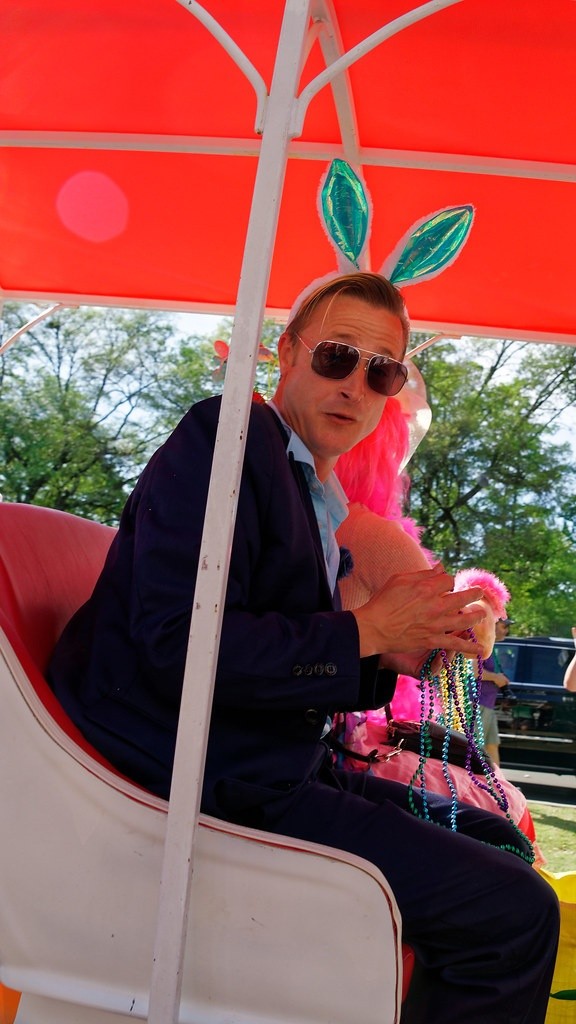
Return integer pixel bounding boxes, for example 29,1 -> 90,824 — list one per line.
293,330 -> 409,396
499,621 -> 509,628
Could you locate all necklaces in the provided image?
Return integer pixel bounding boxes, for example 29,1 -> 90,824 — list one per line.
406,612 -> 536,865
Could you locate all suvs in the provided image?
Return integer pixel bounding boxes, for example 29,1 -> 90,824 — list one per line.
491,636 -> 576,775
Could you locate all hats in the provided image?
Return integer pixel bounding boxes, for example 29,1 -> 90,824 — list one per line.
500,614 -> 515,626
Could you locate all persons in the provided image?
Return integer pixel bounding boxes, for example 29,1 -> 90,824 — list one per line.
564,627 -> 576,692
39,271 -> 561,1024
332,393 -> 544,868
471,615 -> 514,769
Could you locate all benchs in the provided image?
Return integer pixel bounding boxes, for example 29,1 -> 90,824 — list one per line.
0,502 -> 535,1024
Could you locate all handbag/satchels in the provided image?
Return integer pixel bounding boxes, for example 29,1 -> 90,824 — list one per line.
384,718 -> 496,774
